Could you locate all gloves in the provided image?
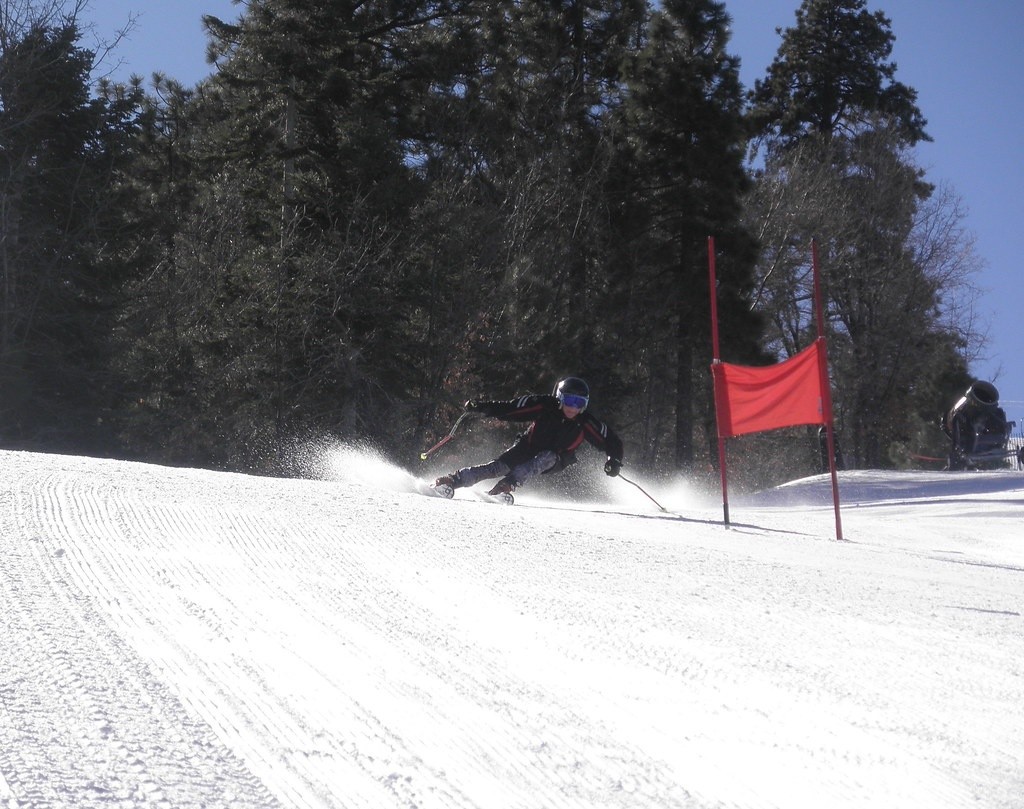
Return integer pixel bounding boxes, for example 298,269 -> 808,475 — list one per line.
604,458 -> 623,477
464,400 -> 479,413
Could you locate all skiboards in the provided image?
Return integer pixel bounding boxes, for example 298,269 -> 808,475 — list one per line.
424,483 -> 516,505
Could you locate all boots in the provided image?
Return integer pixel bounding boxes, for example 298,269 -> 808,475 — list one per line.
436,471 -> 461,499
488,479 -> 514,505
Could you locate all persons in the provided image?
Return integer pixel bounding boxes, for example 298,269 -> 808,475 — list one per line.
432,376 -> 624,504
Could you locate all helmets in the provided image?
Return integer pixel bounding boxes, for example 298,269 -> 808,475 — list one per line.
556,377 -> 589,414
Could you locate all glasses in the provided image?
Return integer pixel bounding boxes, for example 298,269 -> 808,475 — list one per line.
562,393 -> 587,408
